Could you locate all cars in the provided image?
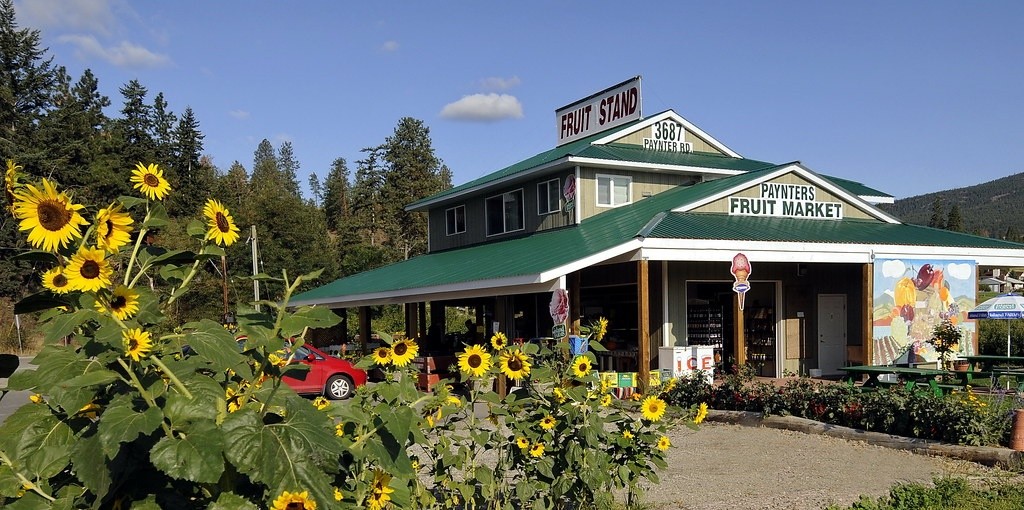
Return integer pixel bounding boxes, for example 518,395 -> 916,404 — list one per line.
233,333 -> 368,402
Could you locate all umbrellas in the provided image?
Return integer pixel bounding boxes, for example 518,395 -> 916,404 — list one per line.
967,293 -> 1024,391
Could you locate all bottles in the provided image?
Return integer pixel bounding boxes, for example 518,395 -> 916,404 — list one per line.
745,306 -> 777,360
687,304 -> 722,350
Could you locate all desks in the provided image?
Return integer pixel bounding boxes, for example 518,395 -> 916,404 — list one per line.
956,354 -> 1024,370
837,365 -> 954,383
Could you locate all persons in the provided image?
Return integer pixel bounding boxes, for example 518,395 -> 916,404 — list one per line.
459,320 -> 482,343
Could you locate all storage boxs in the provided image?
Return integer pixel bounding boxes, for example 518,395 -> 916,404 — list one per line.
597,370 -> 662,389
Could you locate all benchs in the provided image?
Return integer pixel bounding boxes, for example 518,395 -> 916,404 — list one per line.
952,370 -> 1024,394
857,383 -> 964,394
